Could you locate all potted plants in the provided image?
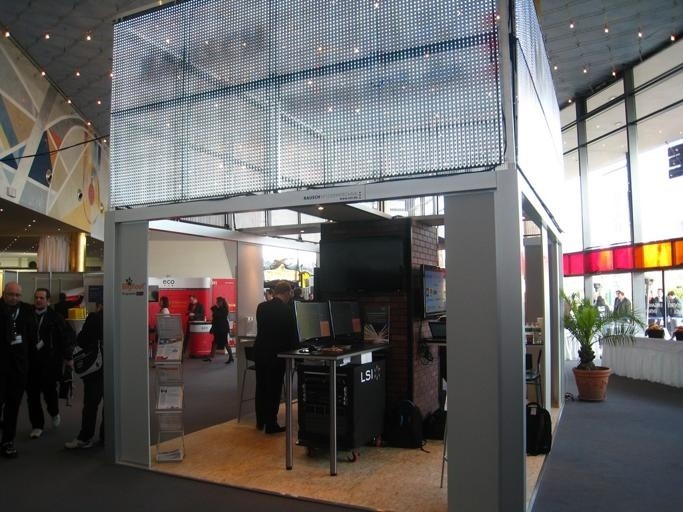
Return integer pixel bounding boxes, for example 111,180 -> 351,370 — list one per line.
559,289 -> 645,401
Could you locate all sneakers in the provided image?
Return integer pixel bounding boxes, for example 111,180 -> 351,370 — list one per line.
52,414 -> 61,427
30,429 -> 42,438
64,434 -> 100,449
6,441 -> 17,454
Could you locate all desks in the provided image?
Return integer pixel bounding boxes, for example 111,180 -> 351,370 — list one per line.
278,340 -> 387,477
599,332 -> 682,388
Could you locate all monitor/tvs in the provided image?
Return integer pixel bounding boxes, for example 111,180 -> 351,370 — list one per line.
330,300 -> 363,342
294,302 -> 332,347
420,264 -> 448,322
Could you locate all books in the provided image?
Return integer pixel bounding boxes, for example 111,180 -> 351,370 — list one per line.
155,315 -> 184,462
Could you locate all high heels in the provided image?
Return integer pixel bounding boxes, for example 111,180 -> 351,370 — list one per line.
203,358 -> 212,362
222,358 -> 234,365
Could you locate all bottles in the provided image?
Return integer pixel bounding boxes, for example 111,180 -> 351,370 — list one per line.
524,321 -> 541,345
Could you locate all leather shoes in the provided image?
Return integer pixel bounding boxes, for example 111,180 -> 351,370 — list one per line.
256,422 -> 287,434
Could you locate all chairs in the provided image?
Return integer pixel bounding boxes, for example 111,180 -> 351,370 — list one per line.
235,345 -> 255,422
421,335 -> 545,489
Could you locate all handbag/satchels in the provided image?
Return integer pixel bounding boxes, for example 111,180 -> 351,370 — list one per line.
72,333 -> 107,377
526,402 -> 551,456
388,400 -> 423,449
423,409 -> 447,440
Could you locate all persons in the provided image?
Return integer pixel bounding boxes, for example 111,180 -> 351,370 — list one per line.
0,281 -> 104,459
148,286 -> 314,365
252,281 -> 300,434
592,288 -> 683,335
